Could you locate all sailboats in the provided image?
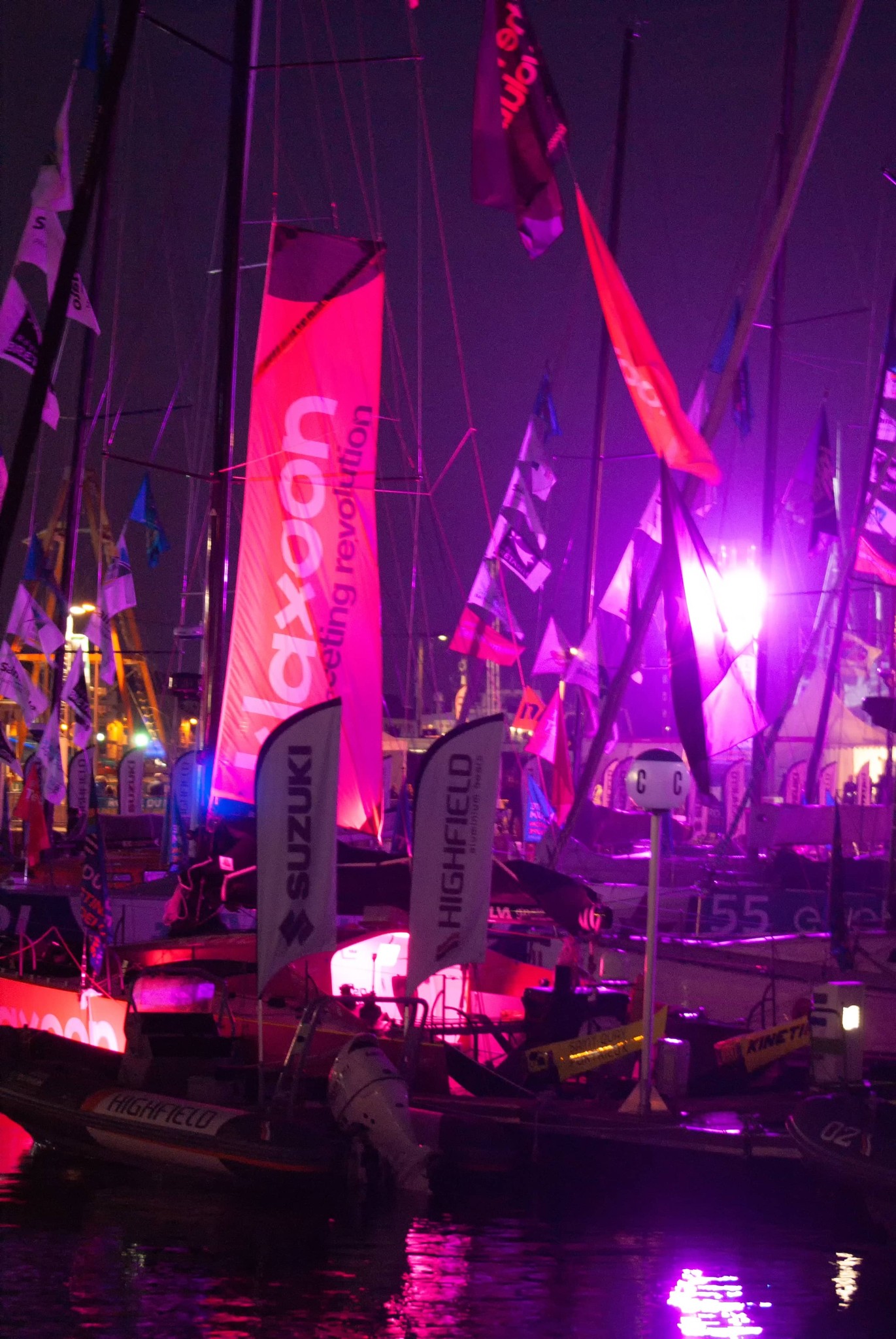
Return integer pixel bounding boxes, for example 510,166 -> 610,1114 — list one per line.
0,0 -> 896,1205
